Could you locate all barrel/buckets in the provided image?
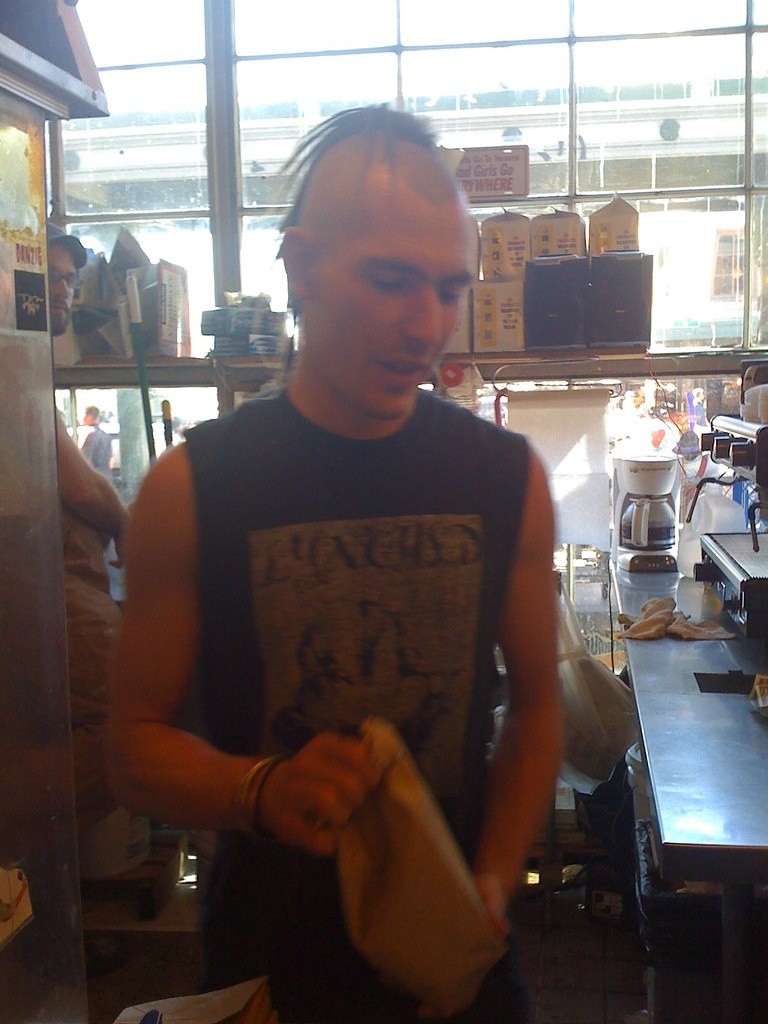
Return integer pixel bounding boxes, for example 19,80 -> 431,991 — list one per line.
610,742 -> 652,839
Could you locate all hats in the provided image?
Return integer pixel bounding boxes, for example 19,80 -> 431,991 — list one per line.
47,222 -> 87,269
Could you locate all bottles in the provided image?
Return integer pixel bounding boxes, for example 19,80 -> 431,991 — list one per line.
676,482 -> 746,579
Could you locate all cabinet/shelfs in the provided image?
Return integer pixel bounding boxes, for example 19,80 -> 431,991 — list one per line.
52,342 -> 768,873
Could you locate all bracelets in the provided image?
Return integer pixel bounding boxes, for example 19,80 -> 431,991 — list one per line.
235,753 -> 290,847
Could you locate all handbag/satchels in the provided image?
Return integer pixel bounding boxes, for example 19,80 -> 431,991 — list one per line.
555,582 -> 640,795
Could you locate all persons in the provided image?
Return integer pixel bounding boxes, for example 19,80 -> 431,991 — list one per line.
81,406 -> 112,486
46,221 -> 127,899
108,105 -> 563,1024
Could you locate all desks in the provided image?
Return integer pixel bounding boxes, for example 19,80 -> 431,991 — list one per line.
608,555 -> 768,1024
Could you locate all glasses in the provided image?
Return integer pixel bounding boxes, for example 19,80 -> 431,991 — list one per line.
48,269 -> 84,290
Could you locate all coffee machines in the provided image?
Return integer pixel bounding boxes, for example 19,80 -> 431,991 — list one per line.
610,456 -> 679,572
